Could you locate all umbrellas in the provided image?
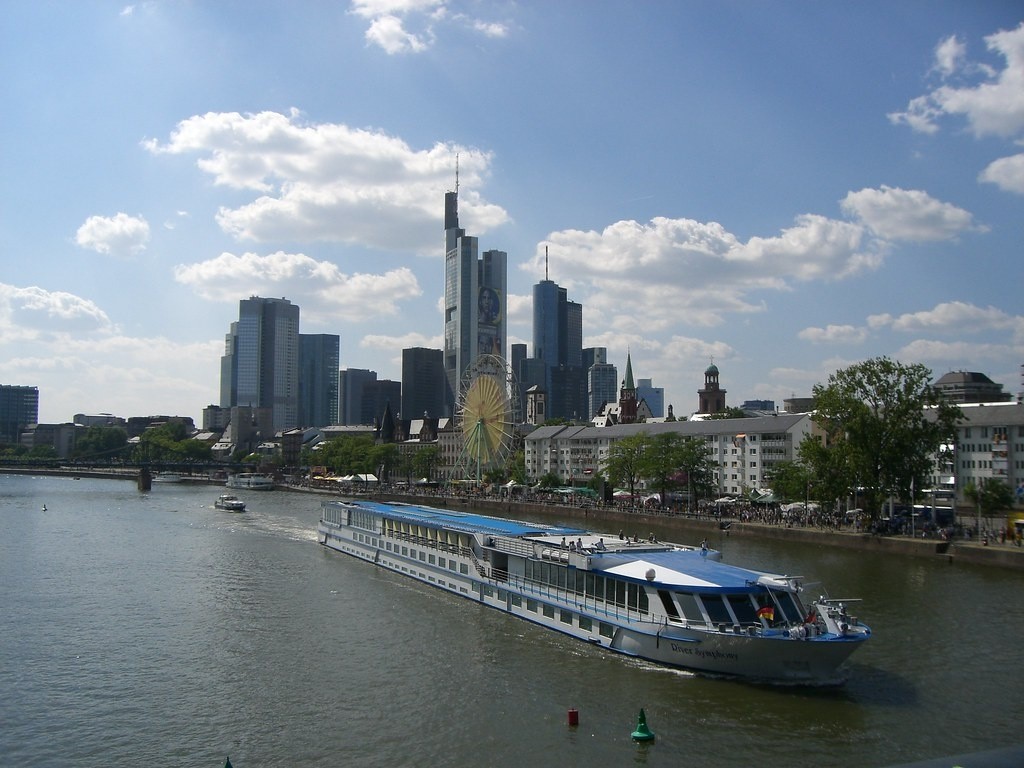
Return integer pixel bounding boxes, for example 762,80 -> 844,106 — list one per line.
780,500 -> 822,512
715,497 -> 742,505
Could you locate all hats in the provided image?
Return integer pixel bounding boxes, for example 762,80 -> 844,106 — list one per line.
578,538 -> 581,540
650,533 -> 653,535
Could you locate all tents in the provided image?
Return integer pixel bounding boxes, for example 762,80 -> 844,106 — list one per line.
312,474 -> 355,486
499,480 -> 528,501
553,488 -> 574,501
418,478 -> 439,487
613,490 -> 640,508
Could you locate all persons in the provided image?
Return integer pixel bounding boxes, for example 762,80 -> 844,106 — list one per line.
698,502 -> 1024,548
385,484 -> 553,502
807,611 -> 817,625
546,495 -> 698,518
561,529 -> 658,553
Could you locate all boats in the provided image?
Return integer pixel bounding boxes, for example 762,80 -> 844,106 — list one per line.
225,473 -> 275,490
214,492 -> 247,511
317,498 -> 873,686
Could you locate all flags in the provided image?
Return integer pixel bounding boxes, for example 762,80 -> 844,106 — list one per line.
755,605 -> 774,620
